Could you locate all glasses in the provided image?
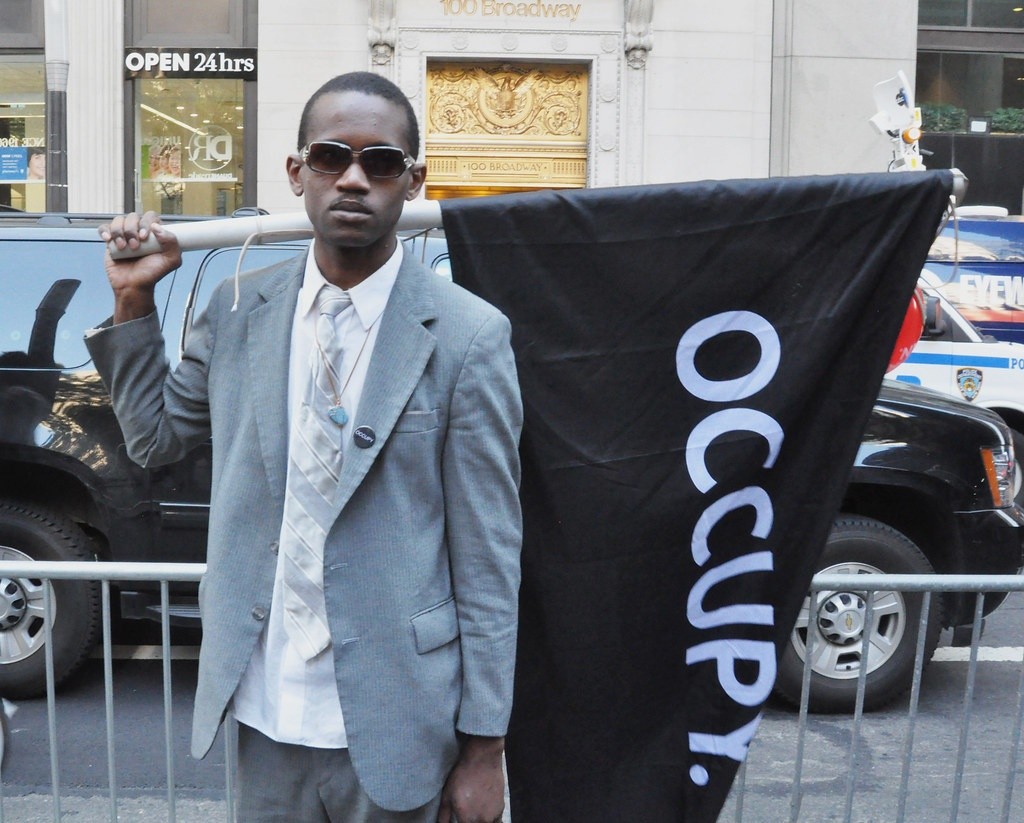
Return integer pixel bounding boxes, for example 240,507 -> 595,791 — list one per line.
300,140 -> 416,180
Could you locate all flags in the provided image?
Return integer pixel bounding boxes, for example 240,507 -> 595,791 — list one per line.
440,170 -> 954,823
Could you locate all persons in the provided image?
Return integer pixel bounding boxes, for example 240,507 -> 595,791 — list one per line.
81,71 -> 525,821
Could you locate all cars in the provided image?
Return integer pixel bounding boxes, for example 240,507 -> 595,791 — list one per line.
870,200 -> 1024,518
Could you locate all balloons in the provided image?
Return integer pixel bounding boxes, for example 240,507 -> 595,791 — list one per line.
886,289 -> 923,375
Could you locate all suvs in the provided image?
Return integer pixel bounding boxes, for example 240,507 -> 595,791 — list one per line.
0,203 -> 1024,706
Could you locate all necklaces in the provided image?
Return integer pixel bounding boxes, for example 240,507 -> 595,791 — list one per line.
313,301 -> 371,429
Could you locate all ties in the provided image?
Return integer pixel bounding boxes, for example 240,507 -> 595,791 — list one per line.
278,288 -> 353,663
